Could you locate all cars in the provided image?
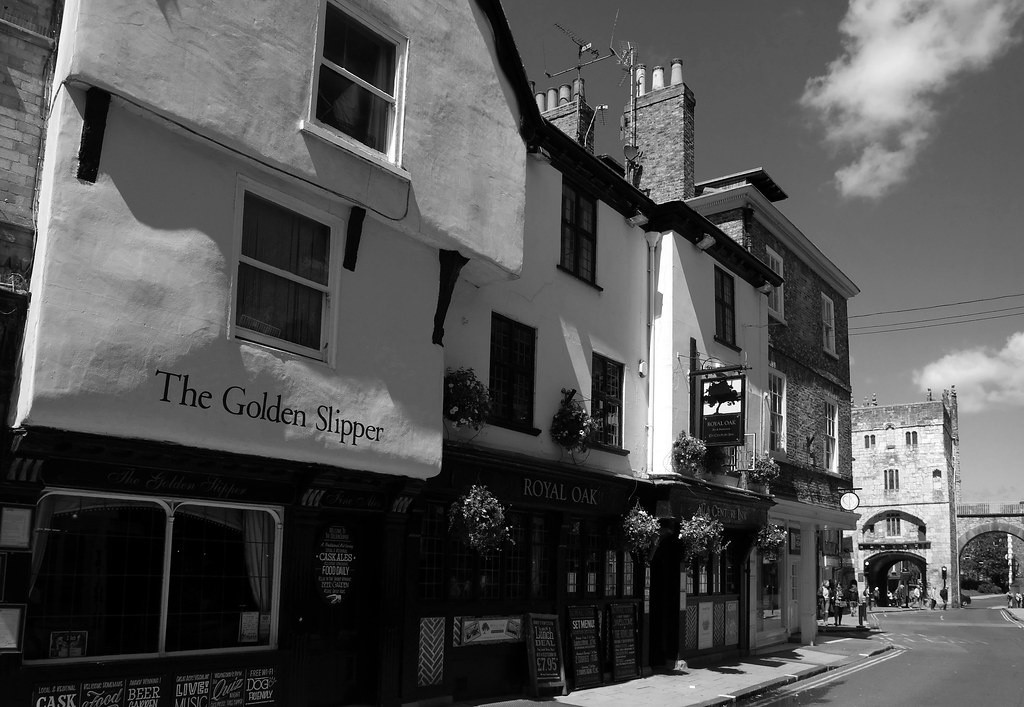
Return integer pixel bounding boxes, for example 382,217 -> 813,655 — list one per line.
961,594 -> 972,607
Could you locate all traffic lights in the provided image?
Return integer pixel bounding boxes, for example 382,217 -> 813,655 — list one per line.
942,566 -> 948,581
864,560 -> 870,577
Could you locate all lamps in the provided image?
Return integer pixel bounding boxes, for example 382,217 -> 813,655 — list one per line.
526,145 -> 551,162
625,210 -> 649,228
755,280 -> 775,294
696,233 -> 717,250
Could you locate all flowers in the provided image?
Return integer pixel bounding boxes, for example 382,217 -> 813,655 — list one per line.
678,509 -> 732,570
674,435 -> 706,475
756,526 -> 788,560
622,507 -> 661,556
456,482 -> 519,556
556,403 -> 602,457
441,368 -> 489,432
747,453 -> 780,484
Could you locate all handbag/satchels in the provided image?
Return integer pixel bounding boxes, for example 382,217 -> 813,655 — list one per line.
839,601 -> 847,608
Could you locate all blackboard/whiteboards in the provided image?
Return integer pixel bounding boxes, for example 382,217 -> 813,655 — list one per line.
564,604 -> 601,687
528,613 -> 564,688
607,603 -> 641,679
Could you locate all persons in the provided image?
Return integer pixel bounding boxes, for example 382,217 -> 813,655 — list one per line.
863,579 -> 926,609
1006,590 -> 1024,608
930,586 -> 938,611
817,579 -> 859,627
941,586 -> 949,610
